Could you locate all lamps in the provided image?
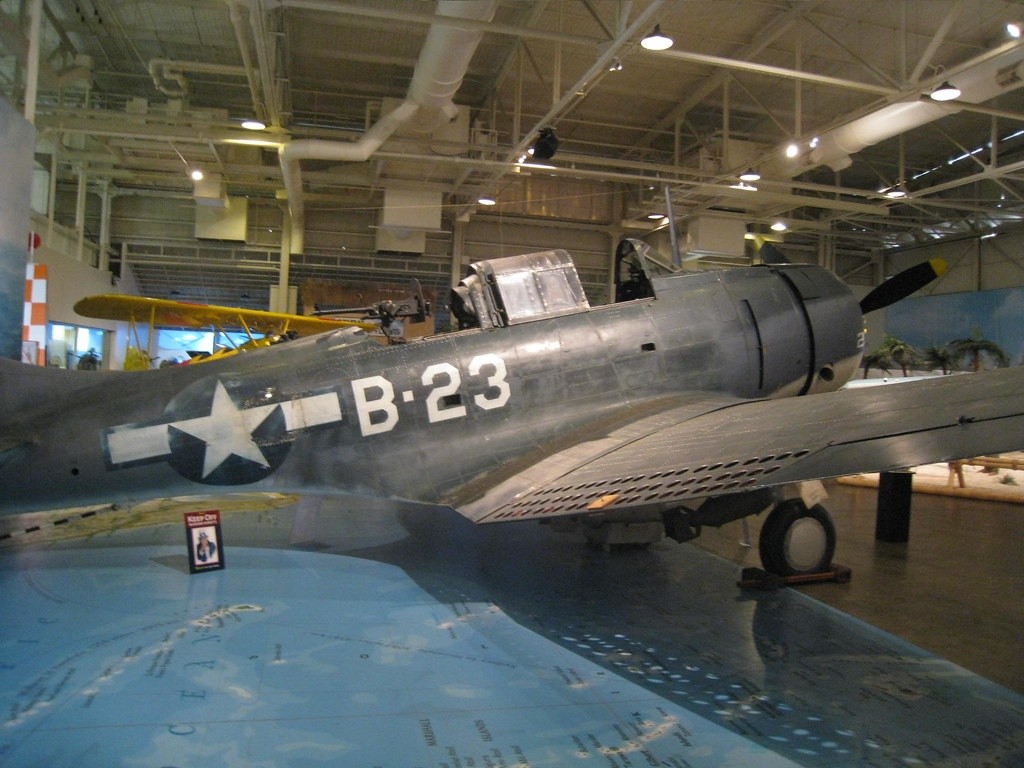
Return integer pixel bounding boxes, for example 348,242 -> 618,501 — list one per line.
770,221 -> 788,234
738,167 -> 761,183
1006,18 -> 1024,38
476,191 -> 497,207
931,79 -> 962,102
886,186 -> 906,198
241,110 -> 267,131
647,213 -> 666,220
532,126 -> 559,159
639,23 -> 673,51
191,168 -> 204,181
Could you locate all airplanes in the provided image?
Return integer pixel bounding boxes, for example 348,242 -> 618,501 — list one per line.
70,294 -> 382,374
0,187 -> 1023,594
